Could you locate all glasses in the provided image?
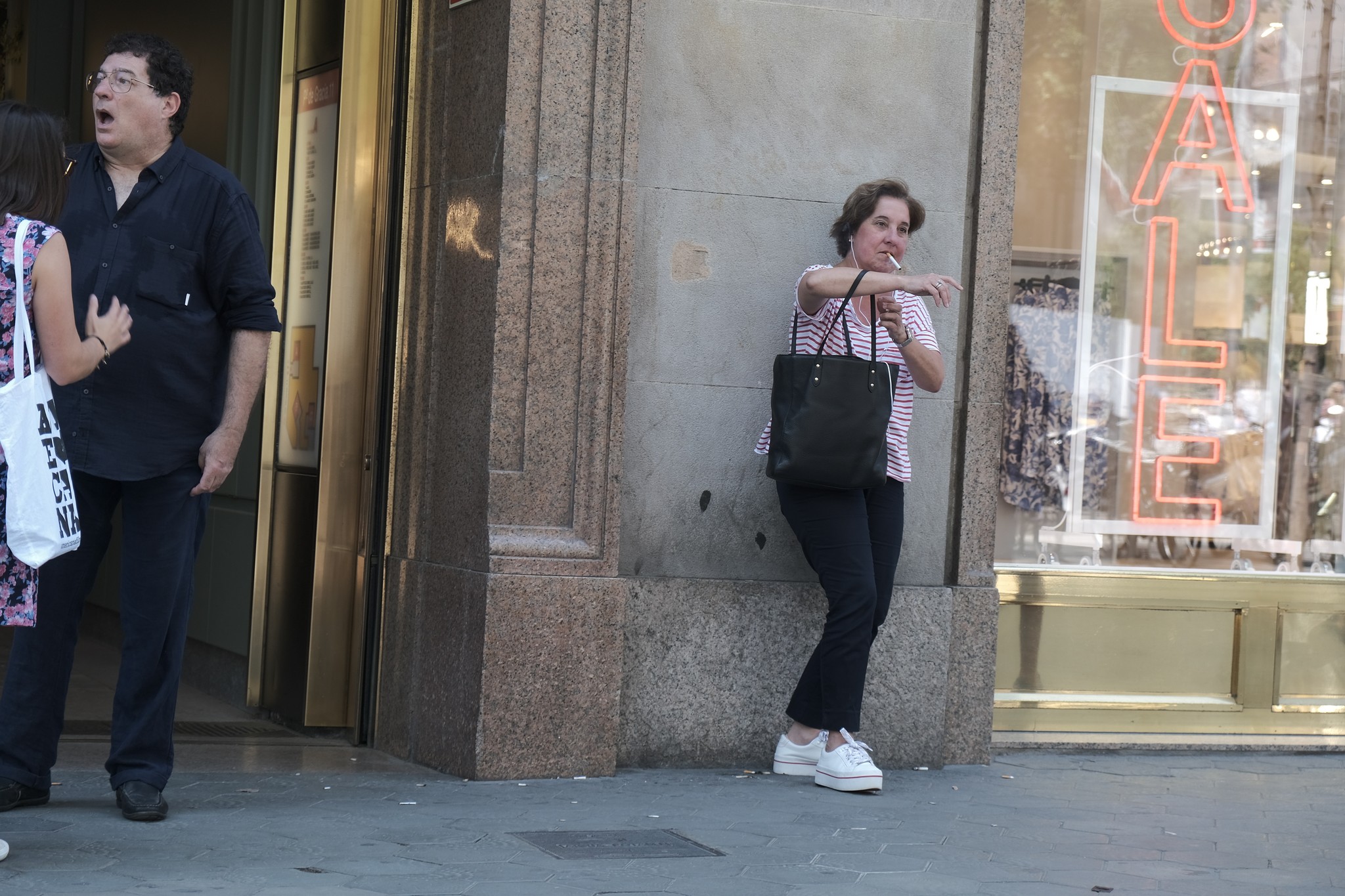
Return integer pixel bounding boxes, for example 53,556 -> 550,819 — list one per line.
86,71 -> 159,94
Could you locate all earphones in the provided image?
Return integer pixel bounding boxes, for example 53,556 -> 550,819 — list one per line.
850,231 -> 853,236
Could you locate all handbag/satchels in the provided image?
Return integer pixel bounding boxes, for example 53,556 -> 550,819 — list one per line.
765,271 -> 900,490
0,219 -> 81,570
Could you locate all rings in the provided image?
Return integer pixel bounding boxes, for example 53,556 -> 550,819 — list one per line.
935,281 -> 944,289
896,309 -> 903,315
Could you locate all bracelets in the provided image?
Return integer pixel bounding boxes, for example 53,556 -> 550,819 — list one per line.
86,333 -> 110,367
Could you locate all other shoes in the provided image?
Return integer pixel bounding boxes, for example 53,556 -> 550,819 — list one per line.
0,777 -> 50,812
0,838 -> 9,859
117,779 -> 169,820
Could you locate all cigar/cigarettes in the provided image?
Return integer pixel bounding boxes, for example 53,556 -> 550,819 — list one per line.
886,252 -> 902,270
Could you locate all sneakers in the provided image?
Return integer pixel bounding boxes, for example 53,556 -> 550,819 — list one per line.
772,731 -> 828,776
815,727 -> 882,792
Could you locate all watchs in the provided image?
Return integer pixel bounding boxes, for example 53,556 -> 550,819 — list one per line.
891,325 -> 915,348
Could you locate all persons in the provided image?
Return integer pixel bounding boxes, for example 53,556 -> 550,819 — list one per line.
1,101 -> 133,862
0,29 -> 282,823
752,178 -> 964,792
